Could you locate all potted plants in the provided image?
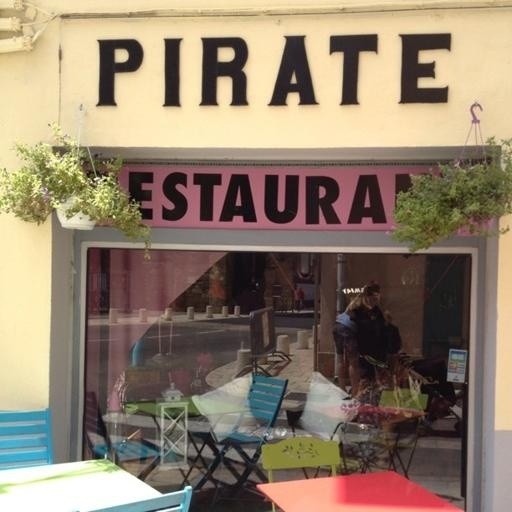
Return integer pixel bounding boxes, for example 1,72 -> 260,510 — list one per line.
390,134 -> 512,256
0,120 -> 152,260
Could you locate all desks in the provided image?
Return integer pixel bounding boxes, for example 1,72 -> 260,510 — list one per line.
0,459 -> 164,512
280,399 -> 429,480
255,470 -> 464,512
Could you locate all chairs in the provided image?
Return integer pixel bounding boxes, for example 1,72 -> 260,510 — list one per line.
0,407 -> 54,470
259,433 -> 342,512
358,389 -> 429,477
85,484 -> 194,512
81,372 -> 289,512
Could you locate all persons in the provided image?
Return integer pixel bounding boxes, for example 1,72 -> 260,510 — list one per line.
332,279 -> 402,380
293,286 -> 307,310
